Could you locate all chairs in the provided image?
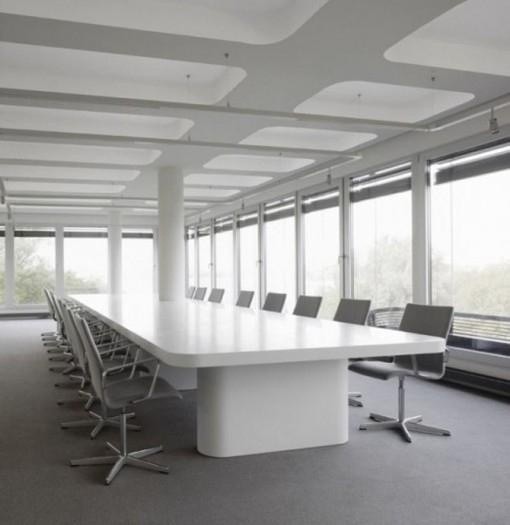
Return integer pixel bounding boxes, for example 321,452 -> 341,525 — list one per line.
185,287 -> 371,408
40,287 -> 183,486
348,304 -> 454,444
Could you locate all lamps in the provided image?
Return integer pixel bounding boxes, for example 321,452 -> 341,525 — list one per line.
488,104 -> 501,139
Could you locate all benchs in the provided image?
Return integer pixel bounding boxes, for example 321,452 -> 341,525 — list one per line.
367,306 -> 510,401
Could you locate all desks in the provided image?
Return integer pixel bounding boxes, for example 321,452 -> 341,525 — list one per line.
65,293 -> 446,459
